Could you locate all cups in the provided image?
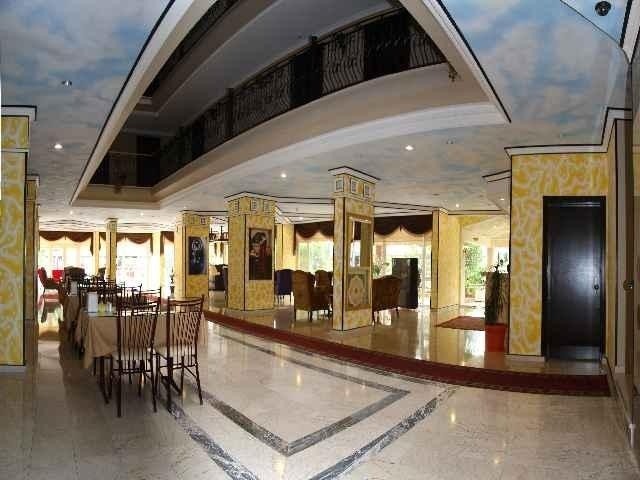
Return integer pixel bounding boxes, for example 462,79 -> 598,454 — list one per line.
107,302 -> 113,313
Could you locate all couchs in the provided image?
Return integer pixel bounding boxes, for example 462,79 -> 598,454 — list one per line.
370,275 -> 404,322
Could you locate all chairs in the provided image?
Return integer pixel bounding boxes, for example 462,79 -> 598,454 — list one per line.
274,268 -> 334,326
38,264 -> 210,422
222,265 -> 229,308
212,265 -> 225,289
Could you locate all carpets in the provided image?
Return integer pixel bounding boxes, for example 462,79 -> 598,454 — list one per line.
434,313 -> 484,332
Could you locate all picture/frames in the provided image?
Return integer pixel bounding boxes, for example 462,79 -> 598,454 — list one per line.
248,226 -> 273,281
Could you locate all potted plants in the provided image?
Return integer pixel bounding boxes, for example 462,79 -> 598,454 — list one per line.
481,260 -> 508,353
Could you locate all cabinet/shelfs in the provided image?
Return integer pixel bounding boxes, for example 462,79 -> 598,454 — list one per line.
392,257 -> 418,312
481,271 -> 508,327
